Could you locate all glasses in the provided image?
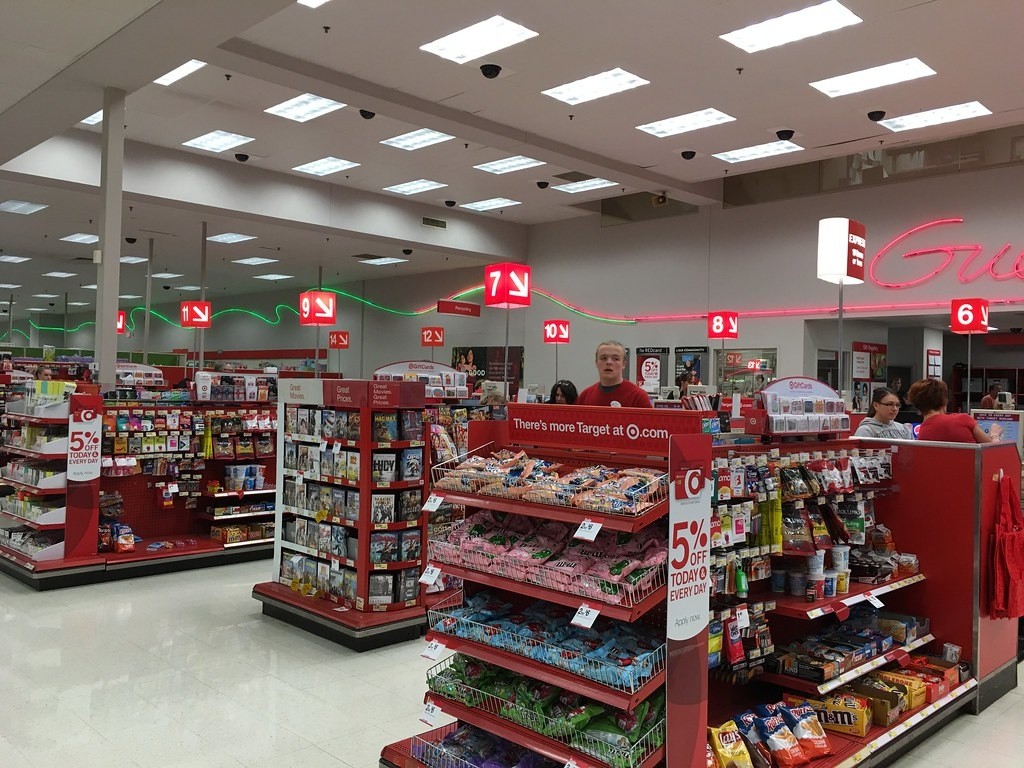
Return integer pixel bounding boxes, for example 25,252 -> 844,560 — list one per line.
876,401 -> 901,408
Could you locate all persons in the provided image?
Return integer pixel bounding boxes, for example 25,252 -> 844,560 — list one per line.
852,382 -> 868,411
572,340 -> 654,408
980,382 -> 1002,410
854,388 -> 915,440
549,379 -> 578,404
891,376 -> 901,391
457,348 -> 476,383
667,373 -> 694,399
907,378 -> 1002,444
35,366 -> 52,380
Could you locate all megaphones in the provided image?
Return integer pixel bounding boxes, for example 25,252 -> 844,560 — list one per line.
651,194 -> 668,208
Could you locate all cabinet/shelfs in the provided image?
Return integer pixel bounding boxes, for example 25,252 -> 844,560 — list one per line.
734,567 -> 937,699
272,402 -> 432,612
0,392 -> 78,572
196,483 -> 278,548
380,490 -> 671,767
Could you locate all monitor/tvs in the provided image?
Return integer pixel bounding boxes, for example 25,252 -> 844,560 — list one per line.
971,409 -> 1024,462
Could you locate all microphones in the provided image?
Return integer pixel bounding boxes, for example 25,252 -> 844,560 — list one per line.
480,64 -> 502,79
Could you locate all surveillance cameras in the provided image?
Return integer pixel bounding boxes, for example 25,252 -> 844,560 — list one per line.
776,130 -> 795,140
3,310 -> 8,313
125,238 -> 137,243
49,303 -> 55,306
403,250 -> 412,255
445,201 -> 456,207
681,151 -> 696,160
868,111 -> 886,121
235,154 -> 249,162
360,109 -> 375,119
163,286 -> 170,290
537,182 -> 549,189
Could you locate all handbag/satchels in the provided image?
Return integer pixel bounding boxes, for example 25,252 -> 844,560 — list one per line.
988,475 -> 1024,620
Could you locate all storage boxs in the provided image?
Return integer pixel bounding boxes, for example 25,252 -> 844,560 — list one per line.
780,643 -> 963,738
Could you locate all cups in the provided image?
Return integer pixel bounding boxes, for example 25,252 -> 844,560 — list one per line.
772,544 -> 851,599
224,464 -> 265,490
